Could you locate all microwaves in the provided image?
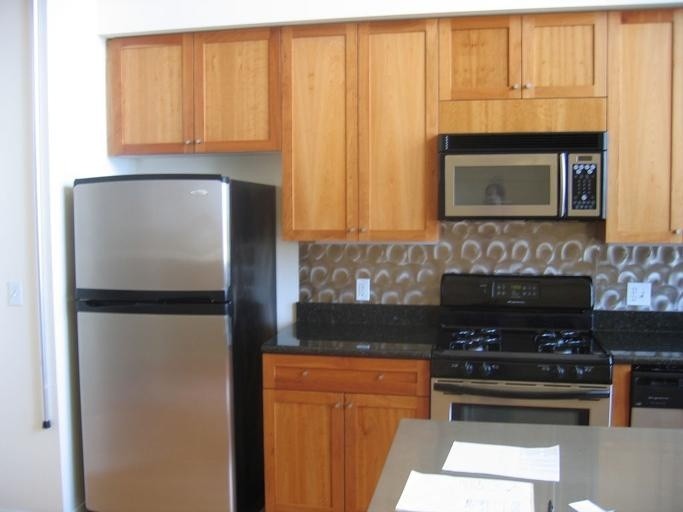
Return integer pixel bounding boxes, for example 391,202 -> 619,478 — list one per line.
438,132 -> 606,221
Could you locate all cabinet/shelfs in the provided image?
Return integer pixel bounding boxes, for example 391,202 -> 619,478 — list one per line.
278,18 -> 440,243
440,12 -> 607,101
256,353 -> 431,512
105,27 -> 282,156
606,5 -> 683,245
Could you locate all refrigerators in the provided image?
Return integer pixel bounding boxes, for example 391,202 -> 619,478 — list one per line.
73,174 -> 276,512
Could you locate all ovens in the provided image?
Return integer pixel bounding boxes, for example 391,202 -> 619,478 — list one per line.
431,379 -> 612,427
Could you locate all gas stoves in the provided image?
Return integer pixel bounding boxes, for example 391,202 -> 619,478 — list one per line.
431,327 -> 608,356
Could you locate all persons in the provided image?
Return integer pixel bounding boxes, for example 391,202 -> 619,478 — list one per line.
481,182 -> 506,207
